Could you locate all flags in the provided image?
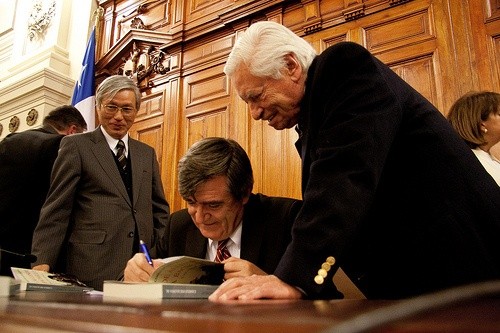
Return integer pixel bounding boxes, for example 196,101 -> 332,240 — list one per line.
71,26 -> 97,132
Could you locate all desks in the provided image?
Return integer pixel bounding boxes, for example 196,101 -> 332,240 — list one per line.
0,291 -> 500,333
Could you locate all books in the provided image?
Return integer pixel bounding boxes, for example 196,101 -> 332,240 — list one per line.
11,267 -> 74,290
101,256 -> 224,300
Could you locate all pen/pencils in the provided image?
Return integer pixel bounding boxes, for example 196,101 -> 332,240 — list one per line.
139,239 -> 153,267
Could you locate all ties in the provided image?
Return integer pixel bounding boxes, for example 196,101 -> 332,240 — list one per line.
116,140 -> 127,171
214,238 -> 231,262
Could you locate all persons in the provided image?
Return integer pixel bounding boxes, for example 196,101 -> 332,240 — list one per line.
30,76 -> 170,290
122,137 -> 344,300
209,21 -> 500,303
0,105 -> 87,278
447,89 -> 500,187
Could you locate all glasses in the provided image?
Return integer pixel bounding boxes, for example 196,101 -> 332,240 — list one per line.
100,104 -> 137,114
